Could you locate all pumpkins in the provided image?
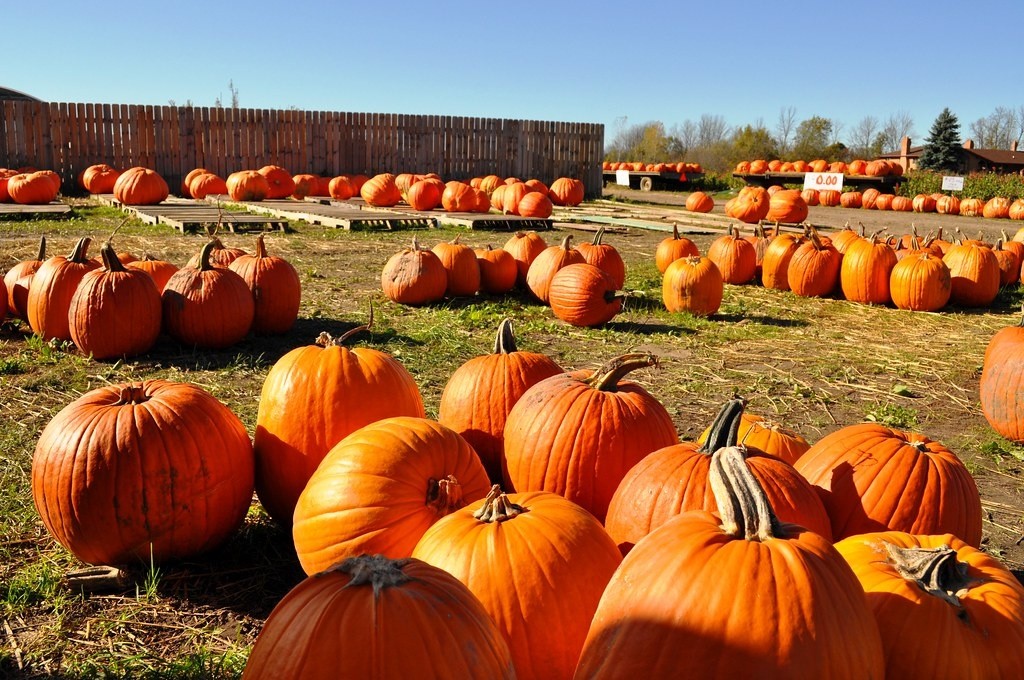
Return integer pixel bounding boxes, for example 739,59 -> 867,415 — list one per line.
601,157 -> 1024,224
0,219 -> 1024,680
0,163 -> 585,219
